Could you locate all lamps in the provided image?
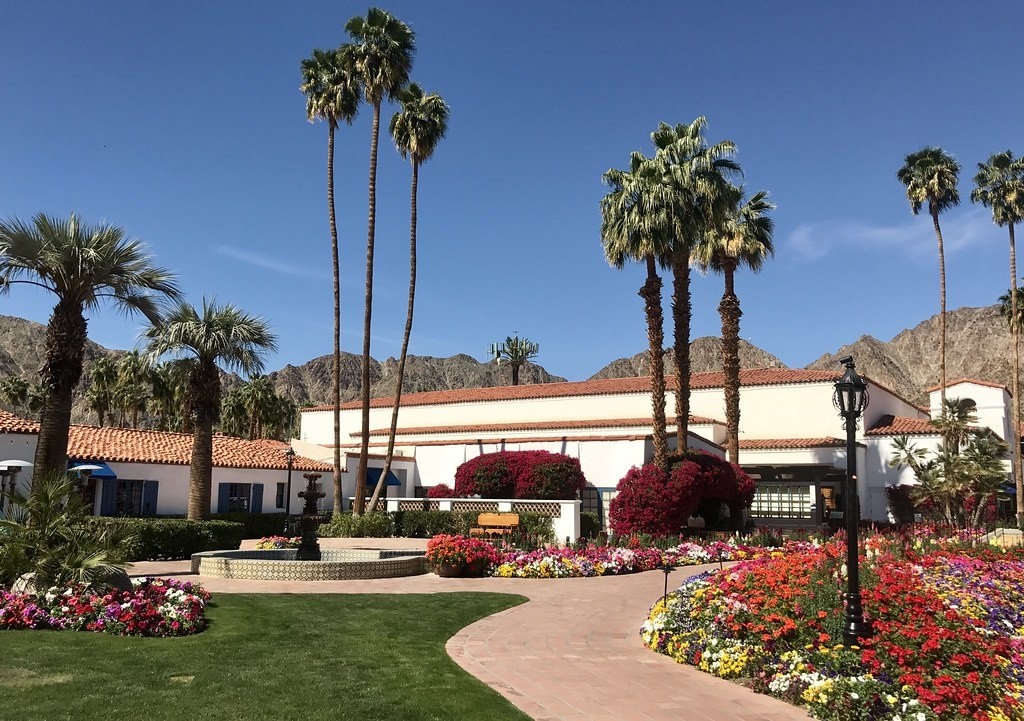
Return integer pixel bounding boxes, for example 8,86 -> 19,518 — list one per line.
716,547 -> 724,556
68,465 -> 103,489
0,459 -> 34,485
657,563 -> 678,574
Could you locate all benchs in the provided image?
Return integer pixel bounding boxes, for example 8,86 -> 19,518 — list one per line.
469,512 -> 519,543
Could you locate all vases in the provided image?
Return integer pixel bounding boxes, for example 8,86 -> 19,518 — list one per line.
436,559 -> 485,578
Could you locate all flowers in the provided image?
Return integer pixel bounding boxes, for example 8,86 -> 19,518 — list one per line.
424,534 -> 504,569
